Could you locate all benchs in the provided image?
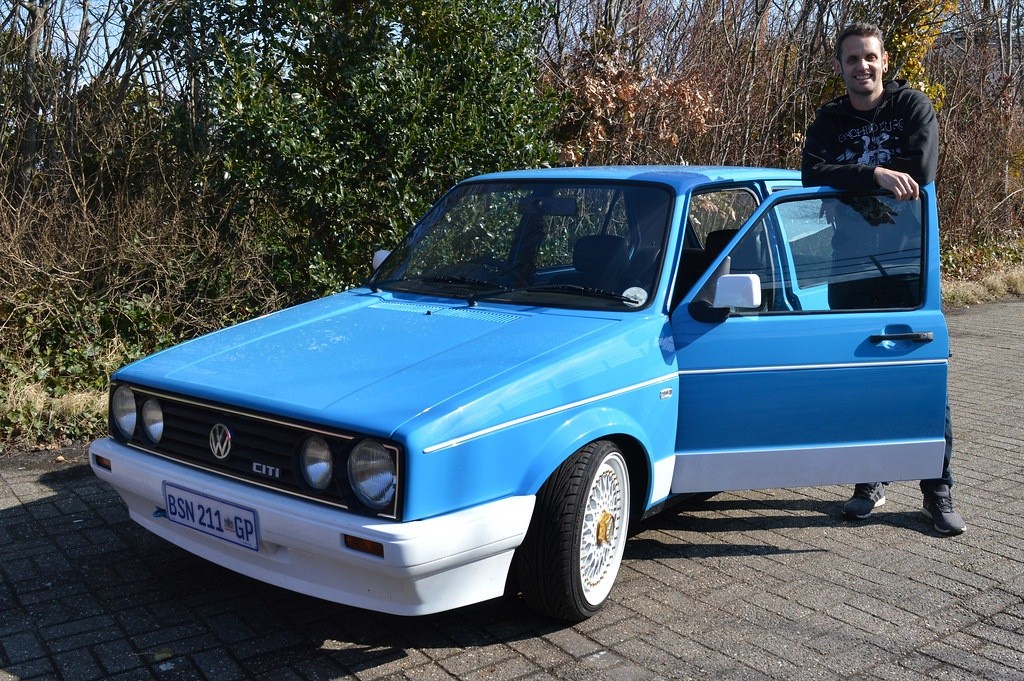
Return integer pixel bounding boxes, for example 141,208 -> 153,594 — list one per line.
631,245 -> 704,312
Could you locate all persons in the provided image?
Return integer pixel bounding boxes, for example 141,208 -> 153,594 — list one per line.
802,23 -> 967,536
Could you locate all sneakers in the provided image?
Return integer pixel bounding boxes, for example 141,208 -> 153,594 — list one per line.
919,484 -> 967,535
843,482 -> 887,520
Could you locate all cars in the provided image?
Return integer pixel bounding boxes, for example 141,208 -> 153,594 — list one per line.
87,167 -> 949,624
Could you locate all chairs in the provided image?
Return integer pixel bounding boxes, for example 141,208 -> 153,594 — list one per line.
572,234 -> 629,299
704,228 -> 768,313
